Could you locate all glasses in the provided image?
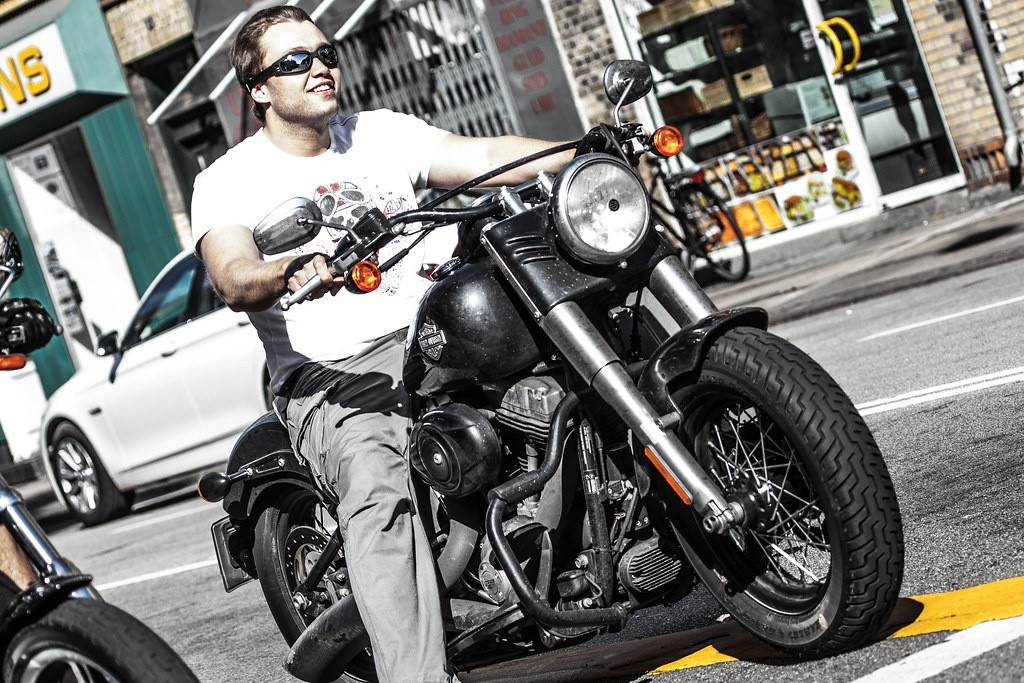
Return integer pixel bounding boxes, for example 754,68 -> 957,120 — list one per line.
246,44 -> 340,87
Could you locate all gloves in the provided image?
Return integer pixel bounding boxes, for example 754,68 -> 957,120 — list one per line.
3,297 -> 56,353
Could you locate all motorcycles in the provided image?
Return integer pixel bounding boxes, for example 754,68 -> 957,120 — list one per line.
0,225 -> 203,683
194,54 -> 906,683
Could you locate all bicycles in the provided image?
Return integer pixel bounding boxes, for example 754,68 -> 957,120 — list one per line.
644,151 -> 752,281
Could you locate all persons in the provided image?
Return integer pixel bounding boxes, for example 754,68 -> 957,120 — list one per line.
191,4 -> 648,683
0,522 -> 38,600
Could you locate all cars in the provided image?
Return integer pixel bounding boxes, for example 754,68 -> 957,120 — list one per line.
39,247 -> 303,528
